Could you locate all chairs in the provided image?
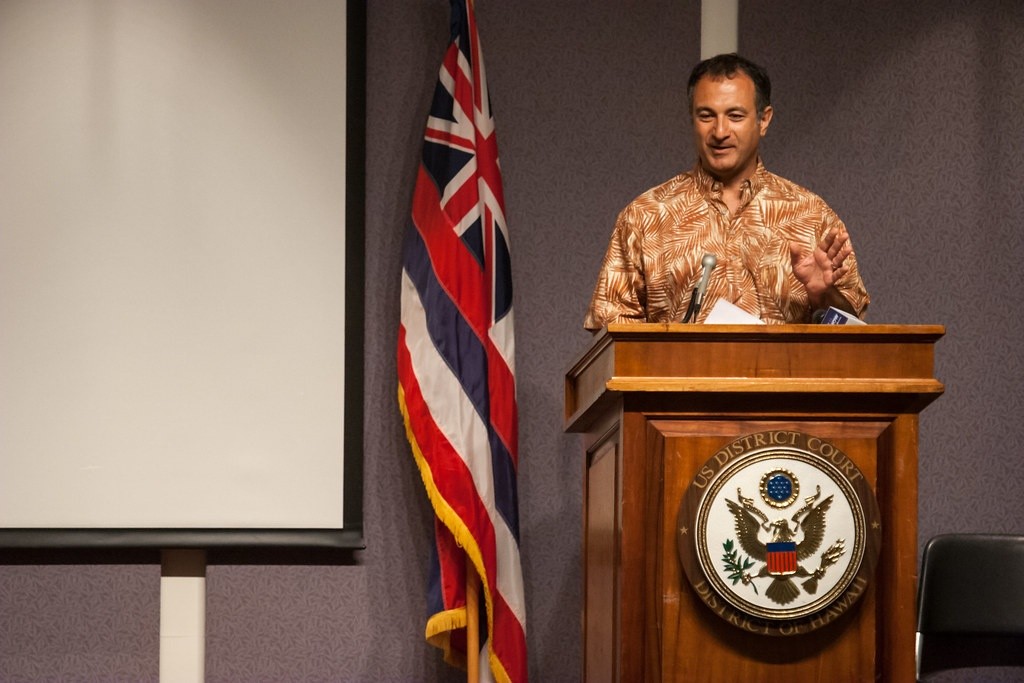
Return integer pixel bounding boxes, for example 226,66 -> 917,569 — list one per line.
915,533 -> 1024,683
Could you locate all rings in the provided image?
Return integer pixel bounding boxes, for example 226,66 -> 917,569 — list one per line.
830,258 -> 838,270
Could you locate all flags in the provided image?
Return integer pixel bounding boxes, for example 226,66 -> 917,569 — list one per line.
390,0 -> 532,682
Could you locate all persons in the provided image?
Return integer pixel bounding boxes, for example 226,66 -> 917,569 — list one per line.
583,53 -> 871,338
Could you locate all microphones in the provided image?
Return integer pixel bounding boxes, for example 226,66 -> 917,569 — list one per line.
694,254 -> 717,314
